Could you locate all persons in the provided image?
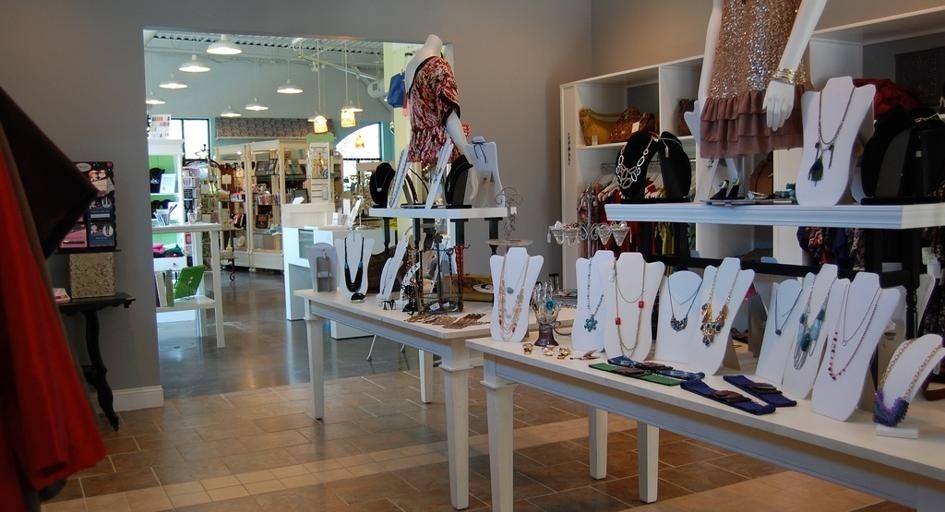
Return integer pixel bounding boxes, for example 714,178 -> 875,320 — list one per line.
696,0 -> 828,159
403,34 -> 469,164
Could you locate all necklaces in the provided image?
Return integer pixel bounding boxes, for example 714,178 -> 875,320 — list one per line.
793,275 -> 838,370
873,337 -> 943,427
614,136 -> 654,188
497,254 -> 530,342
841,284 -> 880,347
343,237 -> 364,293
807,86 -> 856,183
774,289 -> 803,336
613,261 -> 646,358
827,284 -> 882,380
667,277 -> 703,332
583,259 -> 603,332
700,267 -> 741,346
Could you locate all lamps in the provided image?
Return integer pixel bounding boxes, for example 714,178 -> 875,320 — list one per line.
339,39 -> 364,128
220,105 -> 241,118
178,53 -> 211,73
308,40 -> 328,134
277,48 -> 304,94
146,96 -> 166,105
244,97 -> 269,112
157,72 -> 189,90
206,34 -> 242,55
276,55 -> 304,94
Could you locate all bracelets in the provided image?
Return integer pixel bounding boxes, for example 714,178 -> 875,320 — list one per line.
769,66 -> 798,86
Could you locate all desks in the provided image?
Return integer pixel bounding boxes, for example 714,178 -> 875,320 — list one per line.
52,290 -> 134,431
602,200 -> 944,393
292,289 -> 608,511
368,201 -> 516,314
468,323 -> 944,512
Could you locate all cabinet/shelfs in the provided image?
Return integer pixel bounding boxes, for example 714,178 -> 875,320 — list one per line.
153,220 -> 234,348
249,144 -> 284,256
281,142 -> 330,206
215,145 -> 250,256
559,53 -> 712,257
148,136 -> 188,270
804,7 -> 945,274
